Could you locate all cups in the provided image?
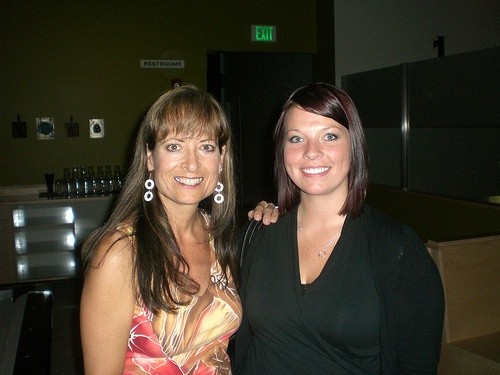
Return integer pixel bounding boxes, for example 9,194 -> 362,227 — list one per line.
44,173 -> 55,193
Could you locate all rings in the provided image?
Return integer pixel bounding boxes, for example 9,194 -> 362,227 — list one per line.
274,206 -> 278,209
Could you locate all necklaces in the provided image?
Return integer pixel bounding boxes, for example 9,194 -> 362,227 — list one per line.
299,225 -> 342,257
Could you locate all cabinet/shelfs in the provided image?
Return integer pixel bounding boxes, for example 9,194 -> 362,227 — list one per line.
0,195 -> 118,285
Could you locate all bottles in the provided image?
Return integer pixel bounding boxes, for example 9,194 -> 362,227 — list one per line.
62,166 -> 123,195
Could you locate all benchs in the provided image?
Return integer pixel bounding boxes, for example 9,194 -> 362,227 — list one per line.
425,237 -> 500,375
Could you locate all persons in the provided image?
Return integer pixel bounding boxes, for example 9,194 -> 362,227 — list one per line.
82,86 -> 280,375
229,83 -> 445,375
